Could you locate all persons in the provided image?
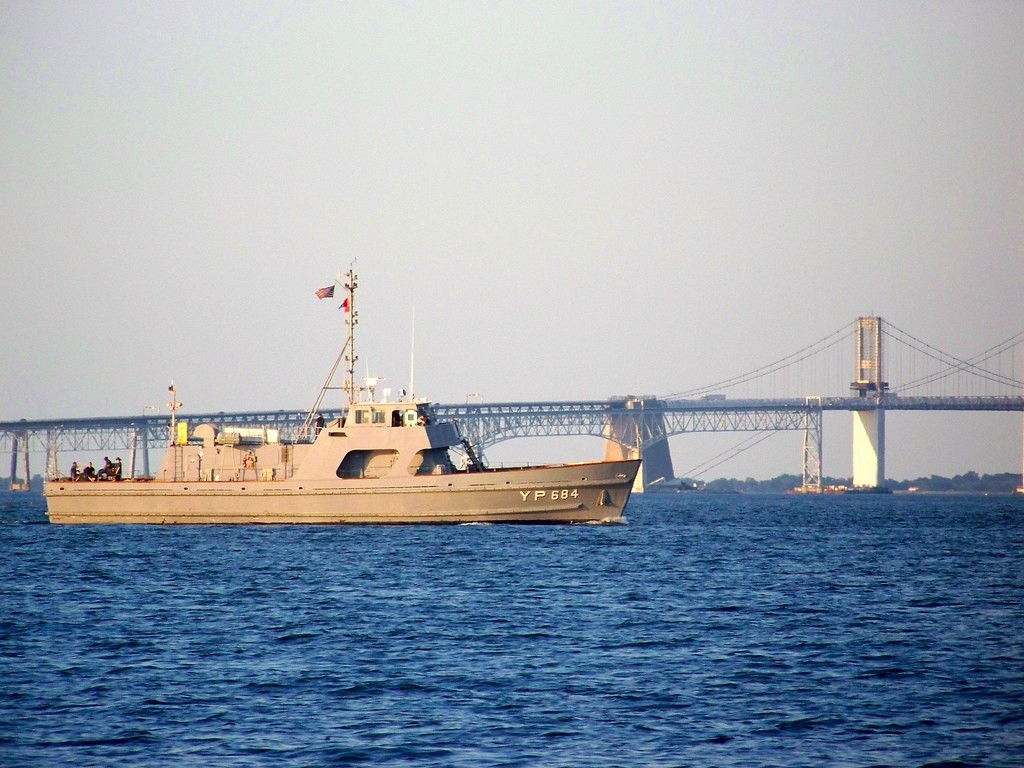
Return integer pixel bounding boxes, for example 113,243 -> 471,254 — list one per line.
315,413 -> 324,440
70,462 -> 80,481
98,457 -> 122,482
83,462 -> 95,483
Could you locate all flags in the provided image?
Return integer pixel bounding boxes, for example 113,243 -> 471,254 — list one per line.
315,285 -> 335,300
339,298 -> 350,313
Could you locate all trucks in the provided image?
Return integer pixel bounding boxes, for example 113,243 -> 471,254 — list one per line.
701,395 -> 726,400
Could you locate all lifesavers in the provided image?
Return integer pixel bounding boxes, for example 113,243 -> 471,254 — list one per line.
243,456 -> 255,468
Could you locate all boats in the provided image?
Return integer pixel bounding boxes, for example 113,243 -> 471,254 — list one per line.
43,268 -> 644,525
